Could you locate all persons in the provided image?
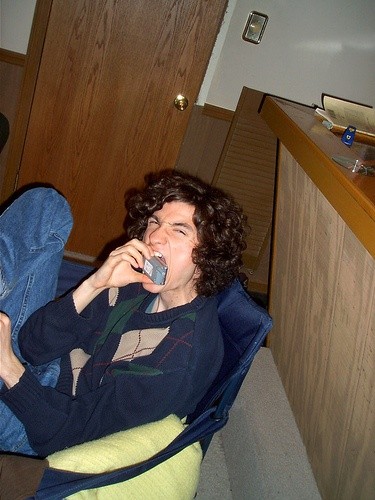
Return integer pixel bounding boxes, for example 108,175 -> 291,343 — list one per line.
0,173 -> 251,458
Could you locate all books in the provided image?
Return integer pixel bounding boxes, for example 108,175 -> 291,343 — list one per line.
313,95 -> 375,137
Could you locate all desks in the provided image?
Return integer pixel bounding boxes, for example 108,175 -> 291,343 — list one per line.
258,94 -> 374,498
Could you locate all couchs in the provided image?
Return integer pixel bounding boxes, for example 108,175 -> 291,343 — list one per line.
194,347 -> 322,498
